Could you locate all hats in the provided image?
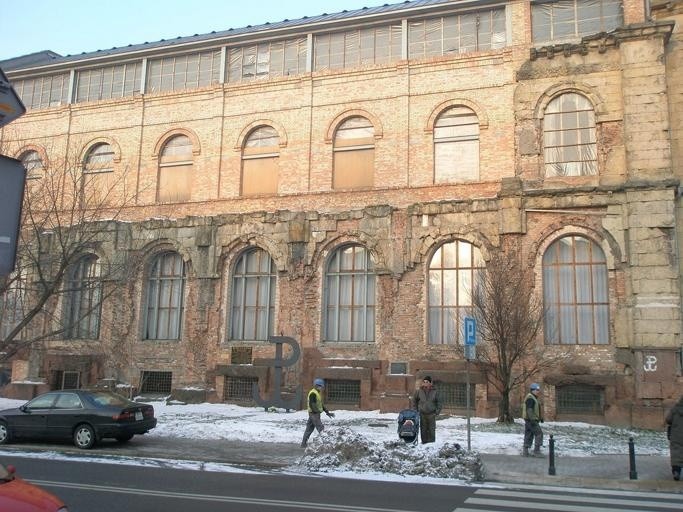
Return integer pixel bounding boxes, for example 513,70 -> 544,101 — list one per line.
424,376 -> 432,383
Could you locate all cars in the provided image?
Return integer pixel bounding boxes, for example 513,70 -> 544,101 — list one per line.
0,387 -> 158,449
0,461 -> 69,512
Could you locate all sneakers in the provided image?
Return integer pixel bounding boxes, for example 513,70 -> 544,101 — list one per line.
673,472 -> 679,481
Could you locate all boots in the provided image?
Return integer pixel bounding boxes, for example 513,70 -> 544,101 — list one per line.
523,448 -> 533,457
533,445 -> 543,455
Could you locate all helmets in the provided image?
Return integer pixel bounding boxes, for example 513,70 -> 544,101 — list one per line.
314,379 -> 325,388
529,383 -> 539,391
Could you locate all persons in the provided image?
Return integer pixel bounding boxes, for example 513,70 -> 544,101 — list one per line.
665,395 -> 683,480
520,383 -> 546,458
412,376 -> 442,444
300,378 -> 335,448
